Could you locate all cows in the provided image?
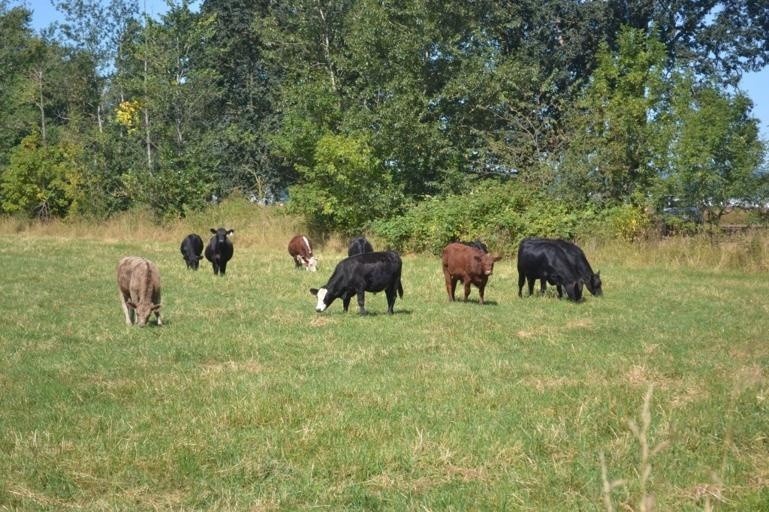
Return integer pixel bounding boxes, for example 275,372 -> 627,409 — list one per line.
309,250 -> 405,316
440,240 -> 504,304
179,232 -> 204,272
517,236 -> 607,302
288,232 -> 319,273
204,227 -> 235,276
343,236 -> 373,258
116,255 -> 164,330
